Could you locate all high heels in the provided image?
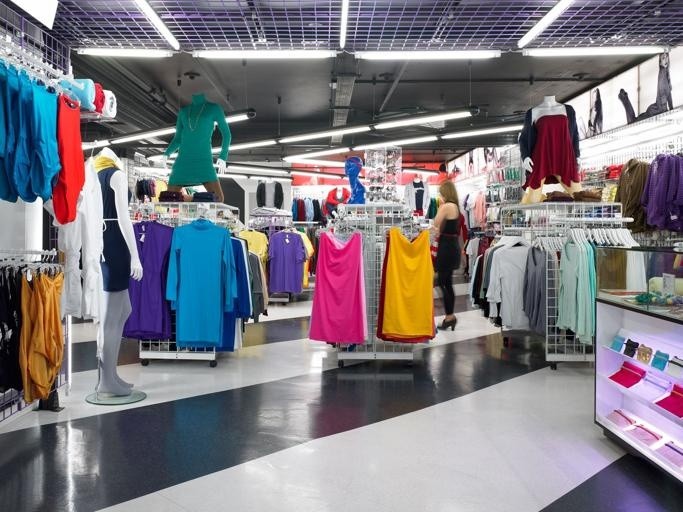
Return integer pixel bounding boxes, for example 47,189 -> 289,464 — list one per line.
437,318 -> 457,330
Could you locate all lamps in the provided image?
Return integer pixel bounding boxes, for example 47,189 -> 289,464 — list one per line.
74,57 -> 528,167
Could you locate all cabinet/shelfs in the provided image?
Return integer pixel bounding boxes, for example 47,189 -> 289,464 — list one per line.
592,296 -> 683,491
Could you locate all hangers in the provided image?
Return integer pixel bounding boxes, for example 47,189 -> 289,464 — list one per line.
0,36 -> 83,107
465,215 -> 641,252
0,247 -> 65,276
125,210 -> 429,240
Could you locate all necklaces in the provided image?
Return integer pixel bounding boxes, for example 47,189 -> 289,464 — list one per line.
186,103 -> 206,132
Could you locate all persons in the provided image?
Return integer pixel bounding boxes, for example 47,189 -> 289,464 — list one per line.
160,90 -> 231,202
85,146 -> 145,399
432,178 -> 464,330
580,88 -> 605,138
516,93 -> 582,191
324,182 -> 351,217
617,53 -> 674,126
409,176 -> 426,213
